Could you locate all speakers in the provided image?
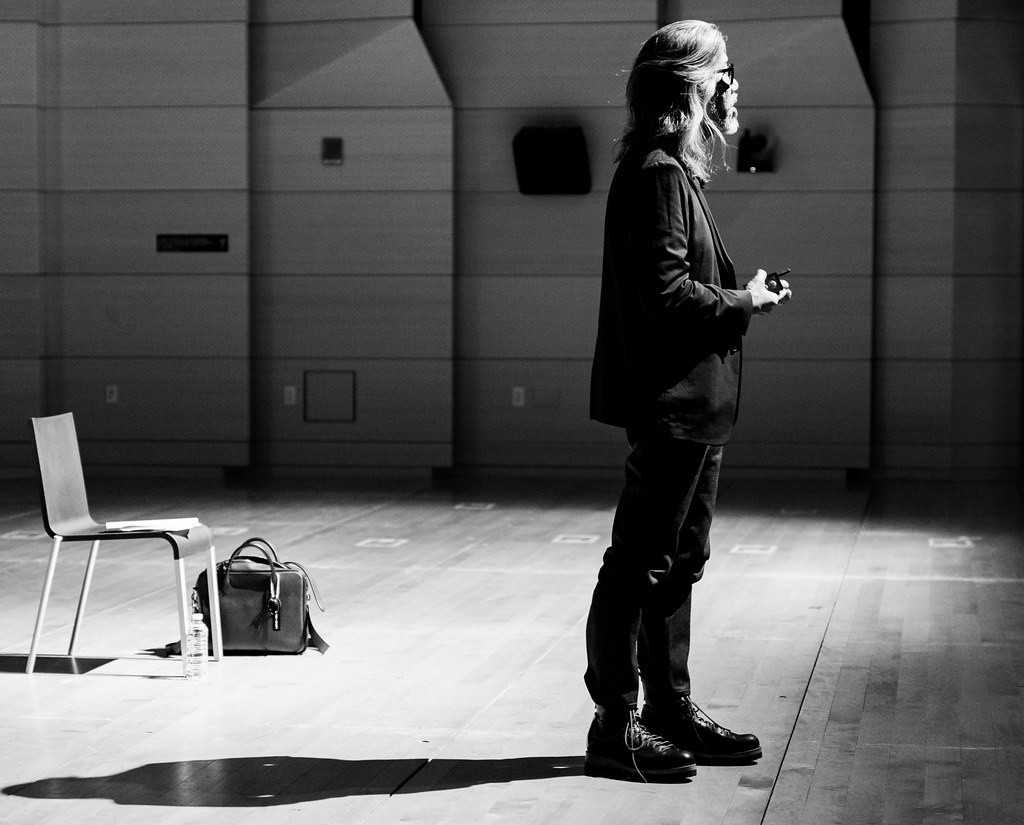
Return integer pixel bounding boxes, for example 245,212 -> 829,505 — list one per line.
513,121 -> 595,196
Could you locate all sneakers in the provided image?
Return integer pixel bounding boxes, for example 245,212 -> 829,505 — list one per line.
582,710 -> 697,779
642,700 -> 762,762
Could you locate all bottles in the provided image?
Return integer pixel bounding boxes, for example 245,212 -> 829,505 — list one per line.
185,613 -> 209,681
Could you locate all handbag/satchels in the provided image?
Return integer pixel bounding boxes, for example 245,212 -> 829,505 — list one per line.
190,537 -> 331,656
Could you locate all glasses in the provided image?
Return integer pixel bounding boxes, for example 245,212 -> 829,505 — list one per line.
713,63 -> 736,83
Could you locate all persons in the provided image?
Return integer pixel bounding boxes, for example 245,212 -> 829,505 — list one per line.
582,15 -> 791,784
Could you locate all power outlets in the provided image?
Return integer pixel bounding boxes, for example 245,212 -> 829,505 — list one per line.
512,386 -> 525,407
283,385 -> 297,407
106,384 -> 120,404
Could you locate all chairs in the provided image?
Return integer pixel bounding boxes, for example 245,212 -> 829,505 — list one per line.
24,410 -> 223,677
